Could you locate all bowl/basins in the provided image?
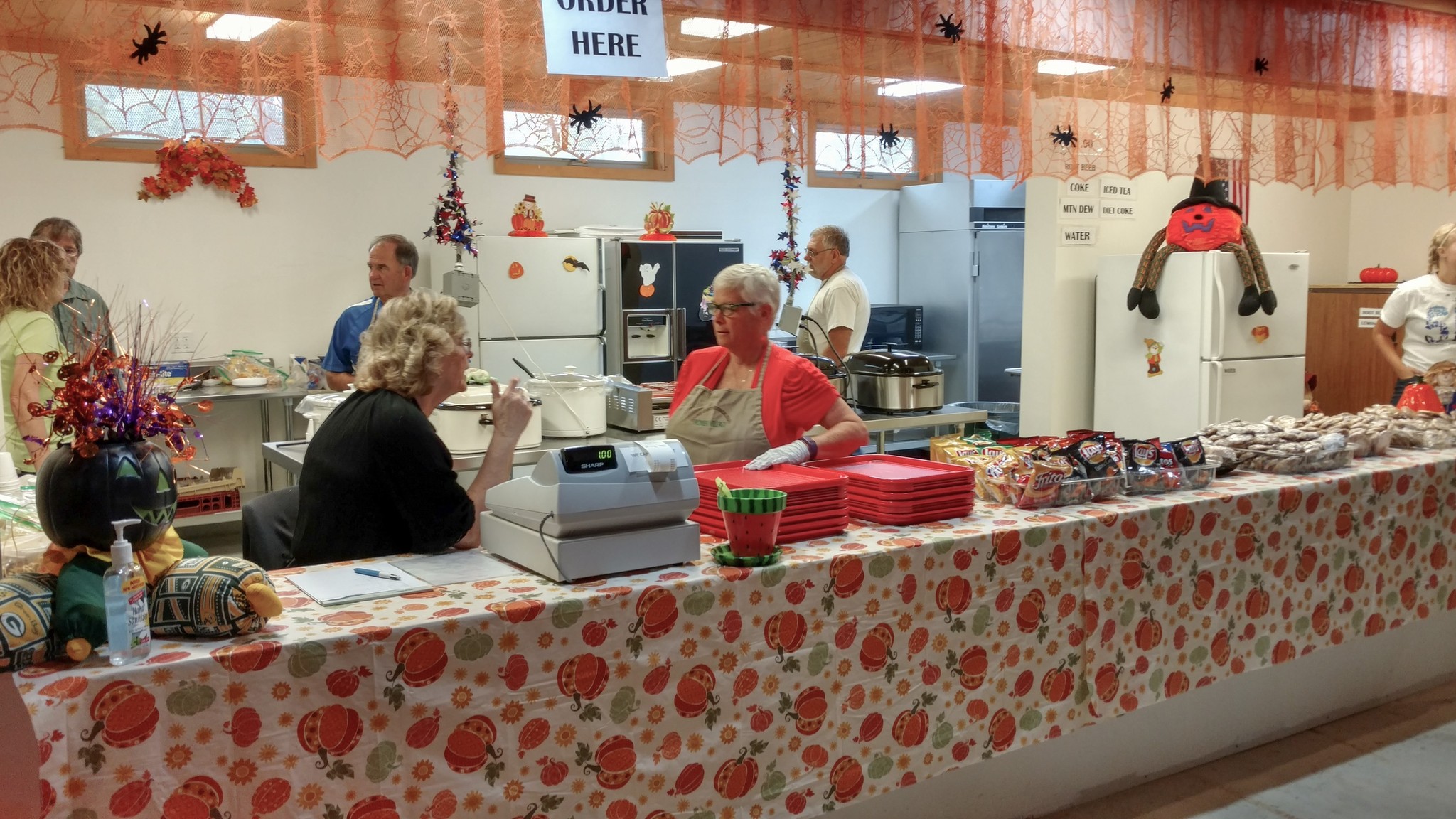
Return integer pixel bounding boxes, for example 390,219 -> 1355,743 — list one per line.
974,459 -> 1248,507
1234,428 -> 1456,475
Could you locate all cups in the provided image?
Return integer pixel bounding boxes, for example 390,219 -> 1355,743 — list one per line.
0,452 -> 21,496
306,419 -> 314,442
27,477 -> 36,486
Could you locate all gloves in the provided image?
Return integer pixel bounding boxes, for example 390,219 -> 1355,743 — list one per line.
744,439 -> 811,471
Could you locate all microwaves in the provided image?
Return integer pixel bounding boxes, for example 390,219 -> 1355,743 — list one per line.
860,303 -> 923,351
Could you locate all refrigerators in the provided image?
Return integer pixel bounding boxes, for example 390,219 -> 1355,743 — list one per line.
605,231 -> 744,384
898,179 -> 1027,406
430,236 -> 603,389
1094,252 -> 1311,441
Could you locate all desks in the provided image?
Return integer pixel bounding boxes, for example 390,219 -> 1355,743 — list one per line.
259,401 -> 988,498
152,376 -> 310,529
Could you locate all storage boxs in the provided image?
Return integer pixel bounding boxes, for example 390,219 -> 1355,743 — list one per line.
1118,453 -> 1222,495
176,464 -> 247,496
983,472 -> 1127,506
137,358 -> 191,386
1237,437 -> 1362,474
174,489 -> 241,519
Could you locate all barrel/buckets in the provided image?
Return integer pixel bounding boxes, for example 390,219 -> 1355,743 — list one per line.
716,488 -> 787,556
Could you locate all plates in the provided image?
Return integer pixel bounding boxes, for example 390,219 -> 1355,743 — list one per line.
232,377 -> 268,388
711,544 -> 784,567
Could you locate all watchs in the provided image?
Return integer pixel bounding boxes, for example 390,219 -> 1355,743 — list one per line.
797,436 -> 817,461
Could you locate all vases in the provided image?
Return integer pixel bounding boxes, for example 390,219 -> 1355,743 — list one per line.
33,435 -> 180,552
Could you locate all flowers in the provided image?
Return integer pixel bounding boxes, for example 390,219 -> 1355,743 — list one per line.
10,288 -> 217,474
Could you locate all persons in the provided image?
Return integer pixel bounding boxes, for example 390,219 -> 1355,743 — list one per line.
292,283 -> 533,567
794,224 -> 871,367
664,263 -> 869,472
0,237 -> 80,477
1371,221 -> 1456,416
321,234 -> 419,392
29,216 -> 127,396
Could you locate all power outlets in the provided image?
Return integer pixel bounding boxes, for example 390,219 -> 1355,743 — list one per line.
168,330 -> 196,354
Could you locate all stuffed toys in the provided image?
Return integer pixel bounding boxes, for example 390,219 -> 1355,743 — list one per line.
1127,176 -> 1277,320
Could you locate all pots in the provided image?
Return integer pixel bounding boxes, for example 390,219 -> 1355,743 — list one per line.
302,383 -> 357,434
428,382 -> 542,454
847,342 -> 944,415
783,346 -> 850,403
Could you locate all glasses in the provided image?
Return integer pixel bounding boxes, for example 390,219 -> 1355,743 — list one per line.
804,248 -> 841,258
706,303 -> 756,317
64,247 -> 79,257
452,339 -> 473,352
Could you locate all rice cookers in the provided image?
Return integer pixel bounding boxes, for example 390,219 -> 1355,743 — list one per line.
527,365 -> 607,438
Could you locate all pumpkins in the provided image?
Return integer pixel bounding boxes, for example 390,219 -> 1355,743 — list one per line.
1360,264 -> 1398,283
1396,375 -> 1447,413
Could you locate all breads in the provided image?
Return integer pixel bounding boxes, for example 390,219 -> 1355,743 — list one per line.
223,357 -> 280,385
